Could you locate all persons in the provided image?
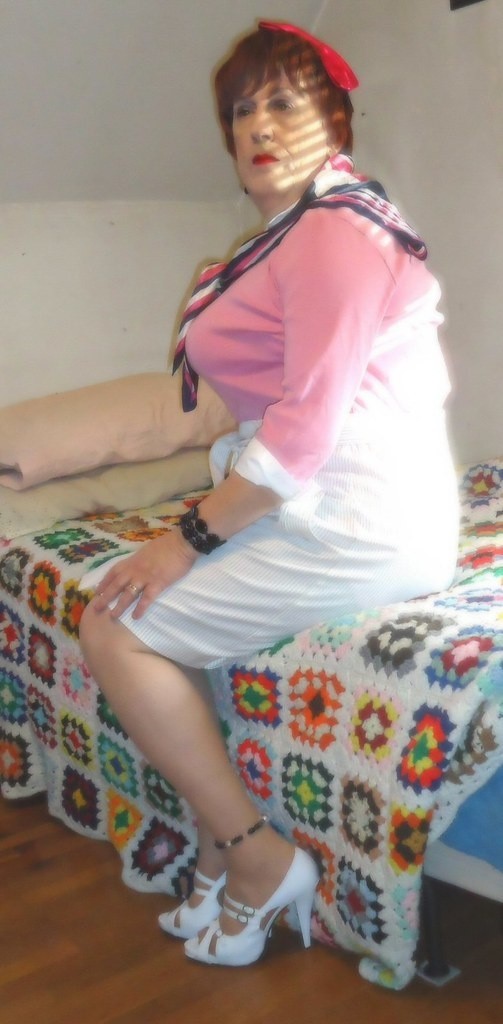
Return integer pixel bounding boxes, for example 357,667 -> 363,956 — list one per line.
78,21 -> 457,965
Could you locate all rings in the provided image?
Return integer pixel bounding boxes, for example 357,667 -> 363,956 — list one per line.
129,584 -> 140,594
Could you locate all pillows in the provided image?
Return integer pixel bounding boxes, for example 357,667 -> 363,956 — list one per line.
0,448 -> 212,541
0,371 -> 237,489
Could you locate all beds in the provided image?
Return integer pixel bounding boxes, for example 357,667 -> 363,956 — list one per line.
0,460 -> 503,989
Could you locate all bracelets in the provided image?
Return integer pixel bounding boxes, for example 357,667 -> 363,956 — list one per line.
180,508 -> 226,555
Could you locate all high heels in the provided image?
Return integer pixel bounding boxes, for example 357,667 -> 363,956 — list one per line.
157,868 -> 227,940
184,845 -> 320,965
